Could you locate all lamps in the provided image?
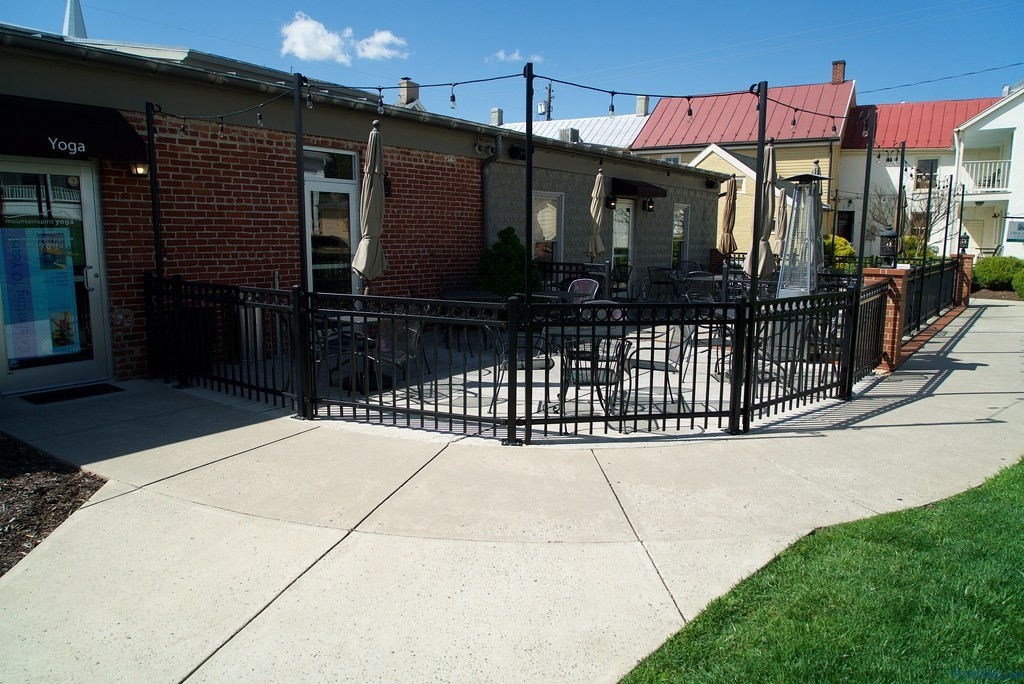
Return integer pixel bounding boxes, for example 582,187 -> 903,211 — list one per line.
450,86 -> 456,109
180,120 -> 186,138
832,117 -> 838,137
647,199 -> 655,212
879,224 -> 897,259
127,153 -> 155,176
608,94 -> 615,121
685,97 -> 693,121
863,121 -> 870,139
756,97 -> 759,119
608,198 -> 618,209
959,232 -> 970,248
306,87 -> 313,110
378,89 -> 384,114
218,117 -> 224,138
875,144 -> 951,190
257,108 -> 264,127
790,110 -> 798,130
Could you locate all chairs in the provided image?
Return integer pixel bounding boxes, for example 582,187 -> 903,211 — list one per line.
809,324 -> 846,363
727,340 -> 787,398
312,314 -> 363,388
612,325 -> 696,415
612,261 -> 783,303
348,318 -> 422,393
554,338 -> 633,413
485,325 -> 554,414
566,278 -> 599,323
686,289 -> 728,338
751,314 -> 811,387
674,324 -> 732,382
579,300 -> 624,339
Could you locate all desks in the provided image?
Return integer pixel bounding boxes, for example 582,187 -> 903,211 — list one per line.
713,308 -> 786,384
327,316 -> 385,395
432,294 -> 508,357
541,325 -> 627,416
516,290 -> 590,354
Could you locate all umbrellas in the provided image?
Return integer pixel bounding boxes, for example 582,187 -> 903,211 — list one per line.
772,185 -> 788,260
352,120 -> 390,295
889,185 -> 907,254
585,168 -> 606,264
742,136 -> 778,284
716,173 -> 738,257
808,158 -> 823,274
